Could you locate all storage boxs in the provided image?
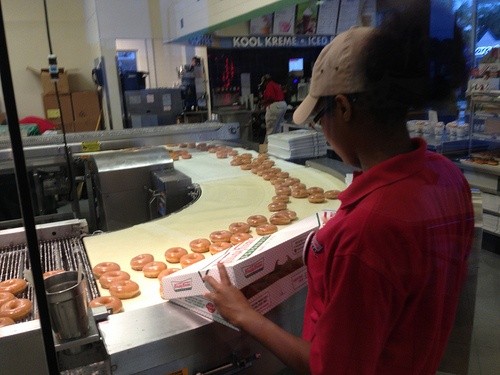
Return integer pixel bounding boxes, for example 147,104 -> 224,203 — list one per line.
466,78 -> 499,97
0,124 -> 42,138
42,91 -> 102,133
26,65 -> 72,95
161,211 -> 338,331
477,62 -> 500,75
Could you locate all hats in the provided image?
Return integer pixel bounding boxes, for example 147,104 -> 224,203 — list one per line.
259,74 -> 271,87
292,26 -> 411,125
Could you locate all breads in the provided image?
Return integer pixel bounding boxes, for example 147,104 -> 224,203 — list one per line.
470,150 -> 500,165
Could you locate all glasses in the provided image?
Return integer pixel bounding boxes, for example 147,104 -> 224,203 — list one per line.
307,93 -> 355,132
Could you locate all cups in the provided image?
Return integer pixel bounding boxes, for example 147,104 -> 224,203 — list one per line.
44,271 -> 90,339
414,120 -> 470,137
302,12 -> 312,30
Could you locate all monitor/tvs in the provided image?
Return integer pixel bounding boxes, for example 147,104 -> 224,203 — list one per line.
289,58 -> 303,72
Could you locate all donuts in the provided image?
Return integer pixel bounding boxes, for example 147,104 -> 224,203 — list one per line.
0,141 -> 341,328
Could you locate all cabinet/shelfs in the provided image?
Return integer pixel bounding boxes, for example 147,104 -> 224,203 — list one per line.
183,109 -> 254,142
454,0 -> 500,235
409,130 -> 490,157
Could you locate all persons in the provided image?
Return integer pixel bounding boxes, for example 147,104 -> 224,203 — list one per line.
260,74 -> 287,143
297,10 -> 315,34
203,25 -> 476,375
